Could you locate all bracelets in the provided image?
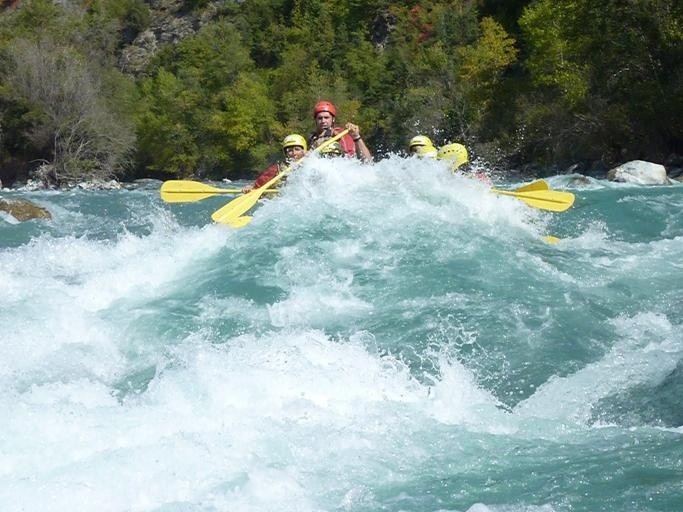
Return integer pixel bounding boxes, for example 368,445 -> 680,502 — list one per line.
352,136 -> 363,143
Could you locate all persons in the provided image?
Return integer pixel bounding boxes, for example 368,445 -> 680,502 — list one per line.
409,134 -> 490,187
320,122 -> 377,169
240,133 -> 307,193
308,101 -> 355,156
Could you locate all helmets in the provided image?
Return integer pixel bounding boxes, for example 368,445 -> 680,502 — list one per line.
282,134 -> 307,154
315,101 -> 336,119
417,145 -> 437,156
436,144 -> 467,169
409,135 -> 432,148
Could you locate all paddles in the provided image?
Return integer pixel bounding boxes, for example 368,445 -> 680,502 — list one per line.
490,179 -> 575,213
160,127 -> 350,229
